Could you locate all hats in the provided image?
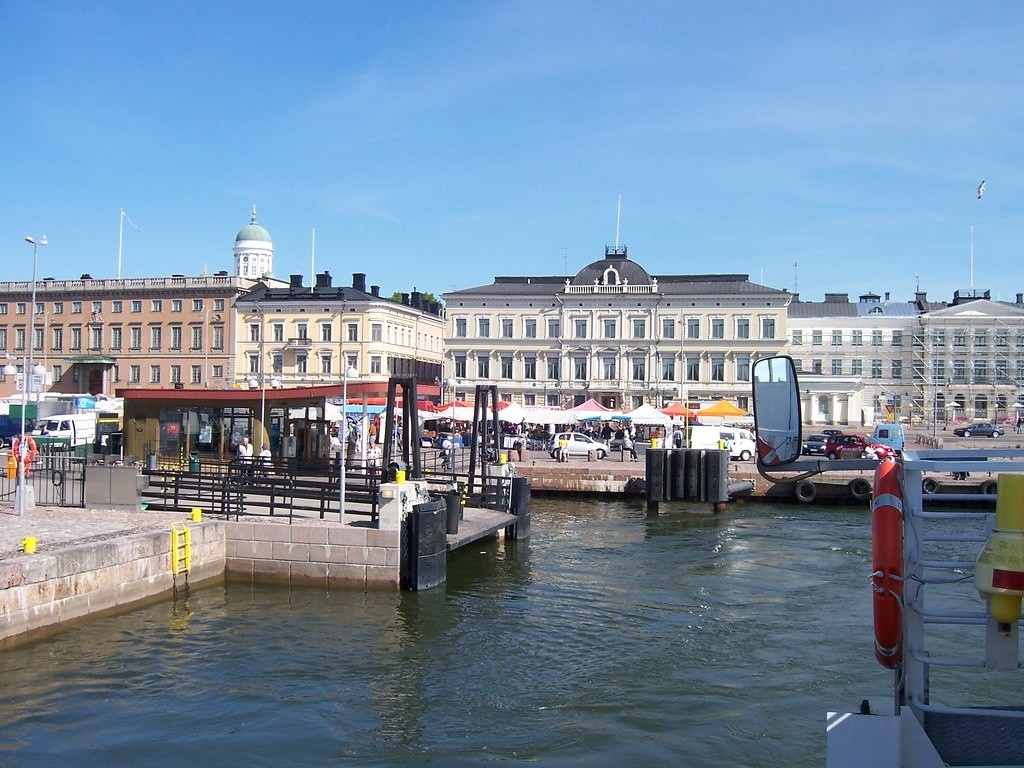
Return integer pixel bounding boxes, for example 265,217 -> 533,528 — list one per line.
447,436 -> 453,440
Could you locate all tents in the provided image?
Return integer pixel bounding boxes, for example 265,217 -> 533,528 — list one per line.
338,398 -> 750,454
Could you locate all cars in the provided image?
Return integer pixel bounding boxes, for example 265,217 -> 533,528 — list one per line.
953,422 -> 1005,438
548,432 -> 610,459
821,430 -> 842,435
802,434 -> 830,455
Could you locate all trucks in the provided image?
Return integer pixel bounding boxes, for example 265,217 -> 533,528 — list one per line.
0,398 -> 38,449
95,402 -> 124,451
32,413 -> 97,448
680,426 -> 757,462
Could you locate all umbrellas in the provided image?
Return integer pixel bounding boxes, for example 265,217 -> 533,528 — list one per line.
290,402 -> 343,422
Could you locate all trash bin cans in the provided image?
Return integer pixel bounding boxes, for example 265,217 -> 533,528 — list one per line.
189,451 -> 200,472
316,434 -> 321,457
281,436 -> 288,458
147,454 -> 156,470
336,452 -> 340,464
446,493 -> 460,534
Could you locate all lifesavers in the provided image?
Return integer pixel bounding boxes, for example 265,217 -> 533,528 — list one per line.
795,480 -> 817,502
14,436 -> 37,463
922,477 -> 939,494
847,478 -> 871,501
757,437 -> 782,465
981,479 -> 998,494
872,455 -> 904,669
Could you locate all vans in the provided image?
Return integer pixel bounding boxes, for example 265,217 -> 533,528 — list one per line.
824,434 -> 896,460
874,423 -> 905,454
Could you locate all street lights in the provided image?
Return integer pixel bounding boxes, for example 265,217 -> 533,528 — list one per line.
447,378 -> 456,480
340,365 -> 358,523
995,404 -> 998,423
909,403 -> 913,426
249,371 -> 278,456
19,236 -> 48,515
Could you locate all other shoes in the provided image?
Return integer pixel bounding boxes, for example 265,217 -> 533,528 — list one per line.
347,469 -> 355,472
630,459 -> 638,462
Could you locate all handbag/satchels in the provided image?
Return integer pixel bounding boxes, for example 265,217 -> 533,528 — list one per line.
439,452 -> 445,459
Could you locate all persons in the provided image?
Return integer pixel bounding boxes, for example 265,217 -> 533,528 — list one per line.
952,472 -> 971,481
723,436 -> 731,461
673,427 -> 683,448
36,424 -> 51,464
223,423 -> 252,458
432,418 -> 660,470
239,438 -> 253,485
329,421 -> 403,475
1016,418 -> 1024,434
258,444 -> 271,487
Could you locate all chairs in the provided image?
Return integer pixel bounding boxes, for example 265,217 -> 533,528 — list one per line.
839,439 -> 845,443
809,437 -> 817,440
851,439 -> 855,443
